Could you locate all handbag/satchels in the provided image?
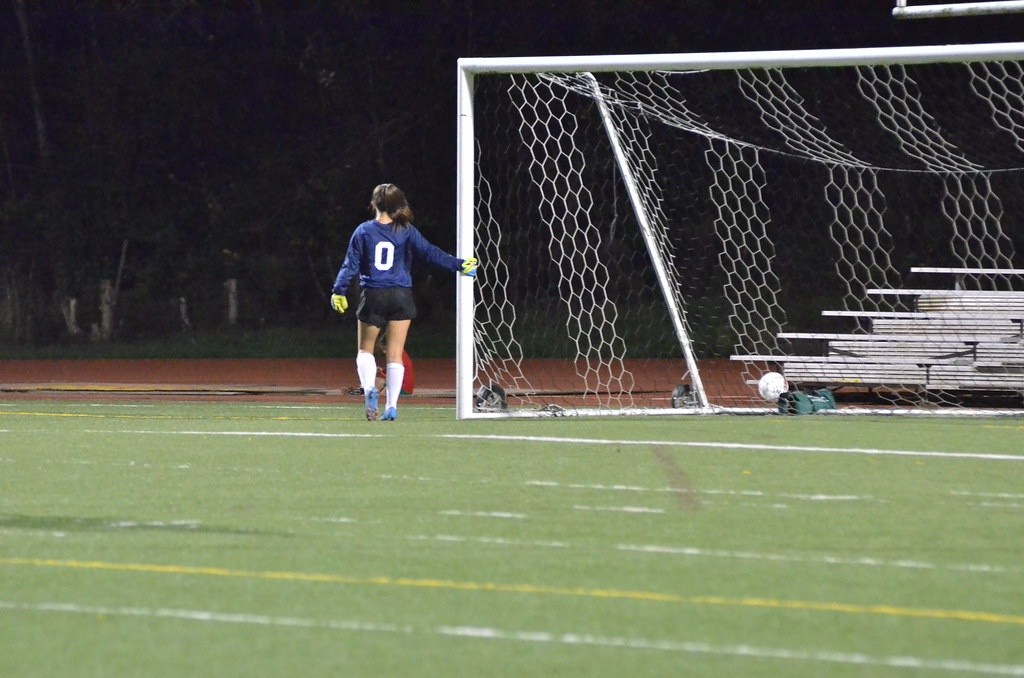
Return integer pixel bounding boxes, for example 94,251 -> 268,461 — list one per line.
778,387 -> 838,415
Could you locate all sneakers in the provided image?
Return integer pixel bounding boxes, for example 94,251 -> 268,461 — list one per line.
380,406 -> 397,421
365,387 -> 379,421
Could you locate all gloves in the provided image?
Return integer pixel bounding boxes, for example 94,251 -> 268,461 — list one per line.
330,293 -> 349,314
461,258 -> 479,278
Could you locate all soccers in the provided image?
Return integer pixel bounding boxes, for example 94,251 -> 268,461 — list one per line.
758,371 -> 789,402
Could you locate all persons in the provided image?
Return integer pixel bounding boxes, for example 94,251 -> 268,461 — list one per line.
331,184 -> 478,421
341,330 -> 414,397
